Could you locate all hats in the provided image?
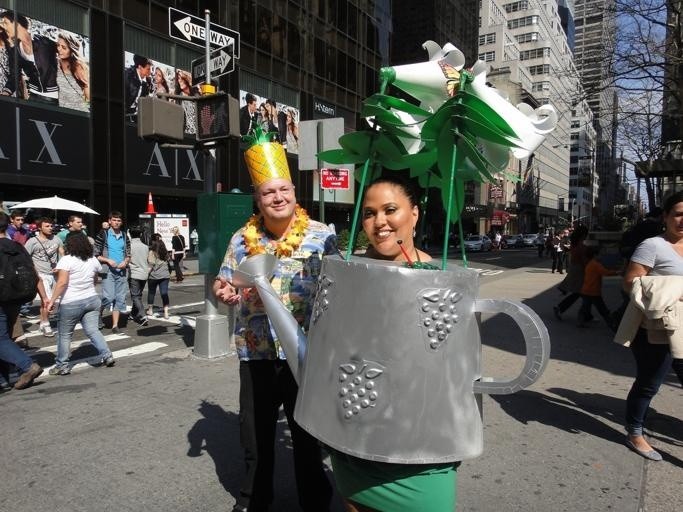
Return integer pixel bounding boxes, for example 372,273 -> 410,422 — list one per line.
240,122 -> 291,189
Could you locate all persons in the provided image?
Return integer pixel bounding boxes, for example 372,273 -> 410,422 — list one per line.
256,103 -> 268,134
239,92 -> 258,137
149,67 -> 170,102
124,54 -> 154,125
54,34 -> 89,113
285,109 -> 299,154
323,175 -> 459,511
613,191 -> 683,462
172,68 -> 195,134
1,208 -> 198,394
212,178 -> 340,510
489,222 -> 617,333
265,99 -> 287,149
0,26 -> 30,100
0,10 -> 61,107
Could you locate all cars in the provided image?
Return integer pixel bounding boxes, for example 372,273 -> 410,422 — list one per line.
504,235 -> 522,249
523,234 -> 543,248
462,234 -> 491,252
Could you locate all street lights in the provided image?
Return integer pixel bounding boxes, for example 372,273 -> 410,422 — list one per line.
552,144 -> 596,233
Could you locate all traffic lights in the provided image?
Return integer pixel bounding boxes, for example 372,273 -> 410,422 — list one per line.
193,93 -> 240,143
136,95 -> 185,143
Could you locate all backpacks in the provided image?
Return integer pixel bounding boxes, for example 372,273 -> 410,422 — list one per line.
0,242 -> 37,305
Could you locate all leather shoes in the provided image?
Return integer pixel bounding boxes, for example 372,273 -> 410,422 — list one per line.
231,504 -> 250,512
624,436 -> 662,461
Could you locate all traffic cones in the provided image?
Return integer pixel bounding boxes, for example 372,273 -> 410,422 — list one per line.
143,192 -> 157,214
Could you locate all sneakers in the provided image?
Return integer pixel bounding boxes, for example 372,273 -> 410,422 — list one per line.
98,309 -> 169,335
553,305 -> 561,321
49,367 -> 69,375
105,357 -> 115,367
40,321 -> 54,337
18,312 -> 37,318
13,363 -> 43,389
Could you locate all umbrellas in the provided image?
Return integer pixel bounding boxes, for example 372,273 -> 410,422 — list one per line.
7,194 -> 99,226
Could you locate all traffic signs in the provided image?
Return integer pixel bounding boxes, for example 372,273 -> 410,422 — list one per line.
168,7 -> 241,61
190,43 -> 235,87
